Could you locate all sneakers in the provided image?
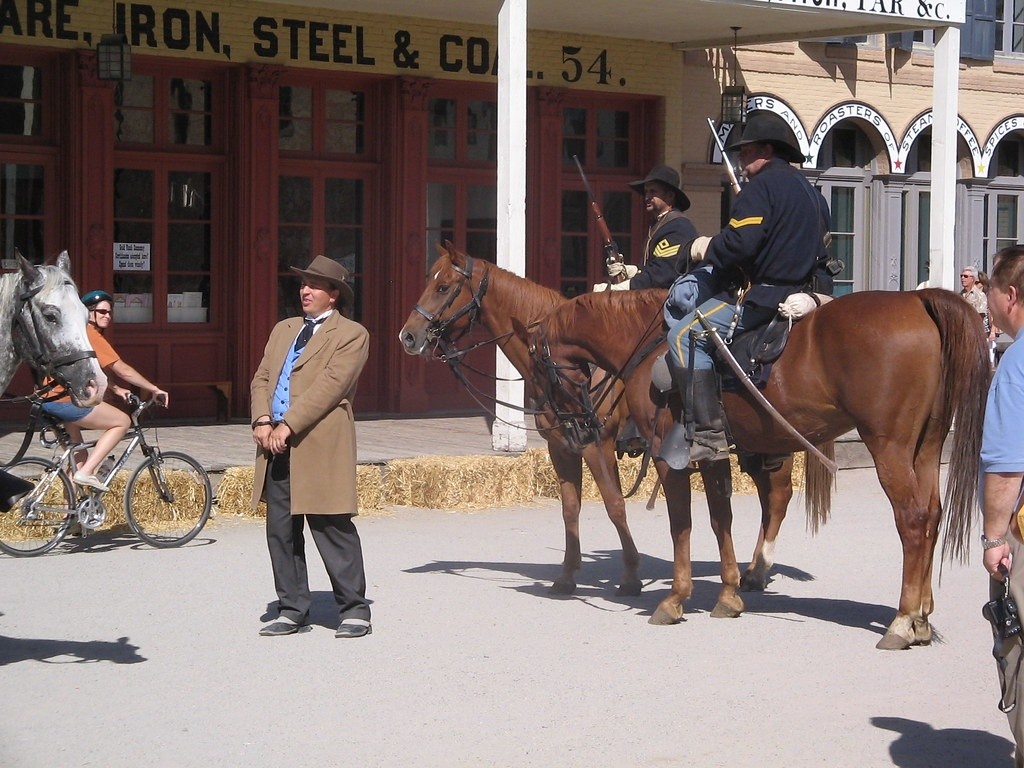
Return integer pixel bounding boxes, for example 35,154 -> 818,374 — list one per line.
74,471 -> 110,493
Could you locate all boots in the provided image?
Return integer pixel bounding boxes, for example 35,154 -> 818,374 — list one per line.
673,365 -> 729,462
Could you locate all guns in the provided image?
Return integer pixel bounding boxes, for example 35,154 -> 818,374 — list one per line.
572,154 -> 629,285
707,115 -> 742,196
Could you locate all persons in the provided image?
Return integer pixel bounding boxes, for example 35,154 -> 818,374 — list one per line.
958,266 -> 1005,372
980,244 -> 1024,768
251,255 -> 374,637
665,109 -> 836,461
33,290 -> 169,536
594,164 -> 698,294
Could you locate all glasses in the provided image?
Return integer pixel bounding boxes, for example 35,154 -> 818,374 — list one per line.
91,308 -> 113,315
975,281 -> 982,285
961,274 -> 973,278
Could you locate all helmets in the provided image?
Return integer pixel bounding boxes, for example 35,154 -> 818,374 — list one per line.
80,290 -> 114,307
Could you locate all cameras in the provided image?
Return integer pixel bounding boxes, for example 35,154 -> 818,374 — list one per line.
982,596 -> 1021,639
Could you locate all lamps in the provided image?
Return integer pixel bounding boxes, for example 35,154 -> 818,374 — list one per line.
95,0 -> 132,83
721,26 -> 748,126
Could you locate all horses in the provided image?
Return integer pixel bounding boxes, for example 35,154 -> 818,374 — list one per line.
512,287 -> 990,648
0,245 -> 108,409
398,240 -> 837,600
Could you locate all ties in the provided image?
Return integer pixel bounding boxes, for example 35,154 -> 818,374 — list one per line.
294,321 -> 317,351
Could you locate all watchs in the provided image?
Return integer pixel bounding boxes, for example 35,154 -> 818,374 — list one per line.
981,535 -> 1008,550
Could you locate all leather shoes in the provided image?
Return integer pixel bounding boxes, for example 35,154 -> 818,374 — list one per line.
259,623 -> 311,634
334,624 -> 372,638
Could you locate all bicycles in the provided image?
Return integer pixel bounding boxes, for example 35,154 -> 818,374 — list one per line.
0,393 -> 212,557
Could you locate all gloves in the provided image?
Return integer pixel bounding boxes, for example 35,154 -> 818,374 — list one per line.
593,279 -> 630,292
606,254 -> 638,279
690,236 -> 712,262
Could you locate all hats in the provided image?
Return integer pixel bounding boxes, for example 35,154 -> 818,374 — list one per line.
724,110 -> 806,164
628,165 -> 690,211
290,255 -> 353,312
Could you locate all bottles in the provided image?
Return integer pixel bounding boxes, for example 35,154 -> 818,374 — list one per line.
88,455 -> 115,494
